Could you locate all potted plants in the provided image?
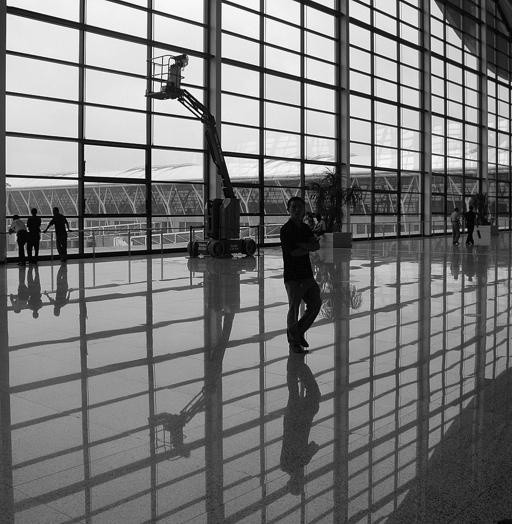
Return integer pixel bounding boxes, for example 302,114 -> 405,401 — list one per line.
313,249 -> 362,323
304,164 -> 364,248
468,192 -> 493,246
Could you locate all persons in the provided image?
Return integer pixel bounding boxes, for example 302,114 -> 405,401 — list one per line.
9,266 -> 29,312
449,206 -> 460,244
312,214 -> 326,241
27,264 -> 43,319
44,260 -> 73,316
461,245 -> 478,281
278,349 -> 323,495
280,196 -> 323,353
9,214 -> 28,266
25,206 -> 42,264
42,206 -> 72,262
462,204 -> 479,246
449,244 -> 461,281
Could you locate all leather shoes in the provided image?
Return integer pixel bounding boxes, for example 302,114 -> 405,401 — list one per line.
290,339 -> 308,353
